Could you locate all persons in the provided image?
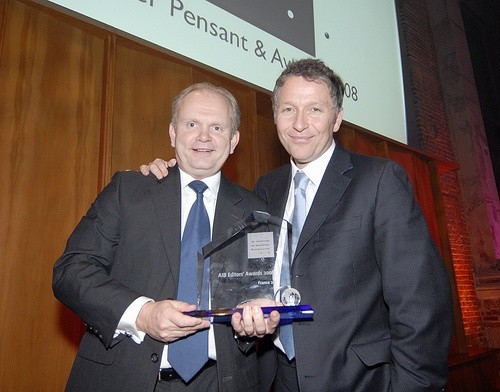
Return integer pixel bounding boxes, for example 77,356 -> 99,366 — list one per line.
52,83 -> 284,392
126,58 -> 453,391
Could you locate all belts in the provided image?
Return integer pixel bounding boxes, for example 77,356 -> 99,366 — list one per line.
157,357 -> 216,382
274,351 -> 299,370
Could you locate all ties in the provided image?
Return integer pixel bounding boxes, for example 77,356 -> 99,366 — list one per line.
166,181 -> 213,385
276,171 -> 311,361
